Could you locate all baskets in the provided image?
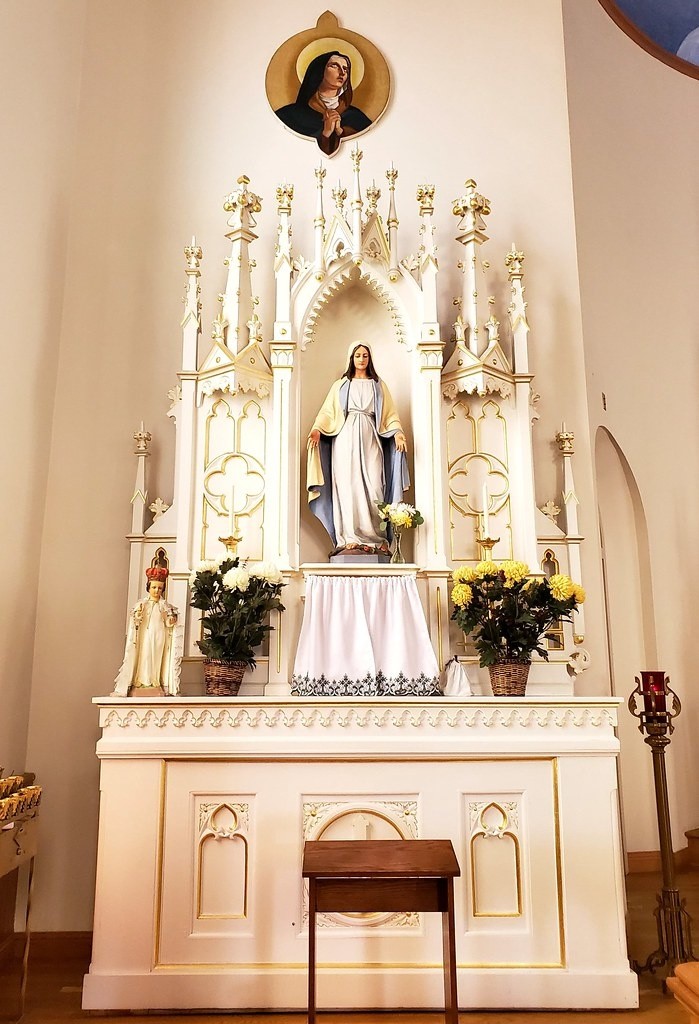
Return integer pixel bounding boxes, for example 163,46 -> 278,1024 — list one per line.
487,659 -> 531,696
203,659 -> 248,696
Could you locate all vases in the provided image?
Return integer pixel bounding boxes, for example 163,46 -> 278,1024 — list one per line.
202,658 -> 247,698
390,533 -> 405,563
488,658 -> 532,695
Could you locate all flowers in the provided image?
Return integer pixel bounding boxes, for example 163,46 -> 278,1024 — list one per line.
373,500 -> 424,563
188,552 -> 290,671
448,558 -> 586,666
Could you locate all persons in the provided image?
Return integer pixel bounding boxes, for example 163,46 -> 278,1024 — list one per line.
130,563 -> 178,688
307,340 -> 408,549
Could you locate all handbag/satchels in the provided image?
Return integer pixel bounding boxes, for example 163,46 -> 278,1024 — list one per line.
440,655 -> 474,696
